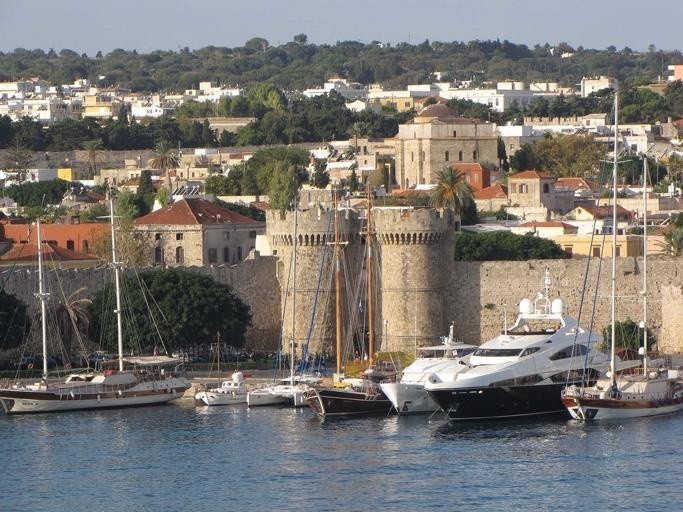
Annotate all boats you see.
[378,264,607,427]
[192,370,249,408]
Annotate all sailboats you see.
[244,186,394,422]
[0,193,192,417]
[558,84,683,424]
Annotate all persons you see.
[64,360,72,370]
[606,368,611,378]
[27,361,33,370]
